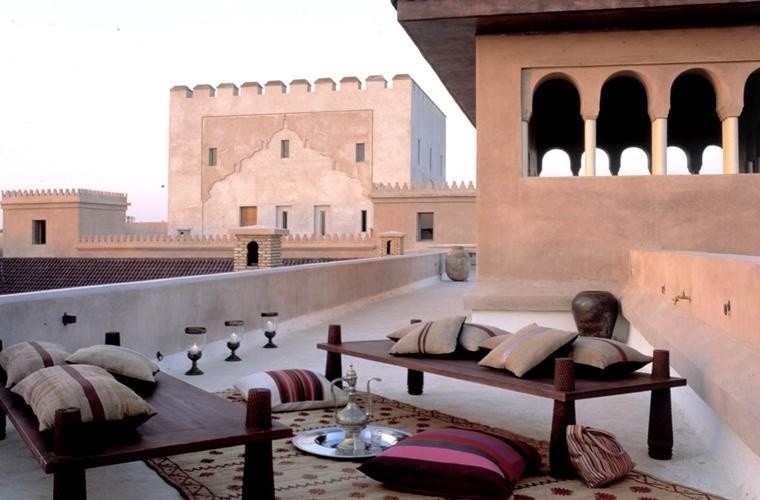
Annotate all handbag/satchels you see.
[566,424,637,488]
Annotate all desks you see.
[317,325,687,480]
[0,332,293,500]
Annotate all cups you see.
[352,431,383,454]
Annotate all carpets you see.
[141,386,728,500]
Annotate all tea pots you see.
[330,364,382,449]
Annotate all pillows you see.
[233,369,349,412]
[477,334,512,350]
[10,364,159,432]
[0,341,72,390]
[64,345,160,386]
[478,323,582,379]
[387,316,467,358]
[458,323,510,352]
[386,321,425,342]
[569,336,654,381]
[355,423,541,500]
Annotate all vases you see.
[568,289,620,339]
